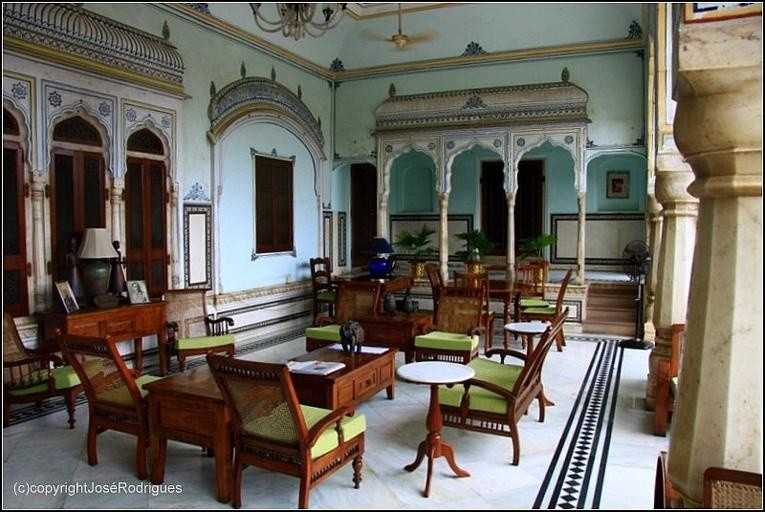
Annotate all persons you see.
[60,285,78,313]
[130,282,149,303]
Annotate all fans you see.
[367,2,442,55]
[618,240,656,350]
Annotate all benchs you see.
[427,306,569,471]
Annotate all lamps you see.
[368,236,393,283]
[80,226,120,294]
[247,3,353,40]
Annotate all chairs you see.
[307,255,577,372]
[53,322,166,481]
[654,323,686,438]
[160,286,236,372]
[203,349,369,505]
[653,447,763,510]
[2,312,108,428]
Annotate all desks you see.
[138,369,238,506]
[32,296,168,390]
[398,360,476,500]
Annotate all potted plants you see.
[520,229,555,281]
[451,228,491,288]
[392,227,442,279]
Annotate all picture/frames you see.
[53,280,80,314]
[125,278,151,305]
[607,169,630,199]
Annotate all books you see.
[284,360,346,376]
